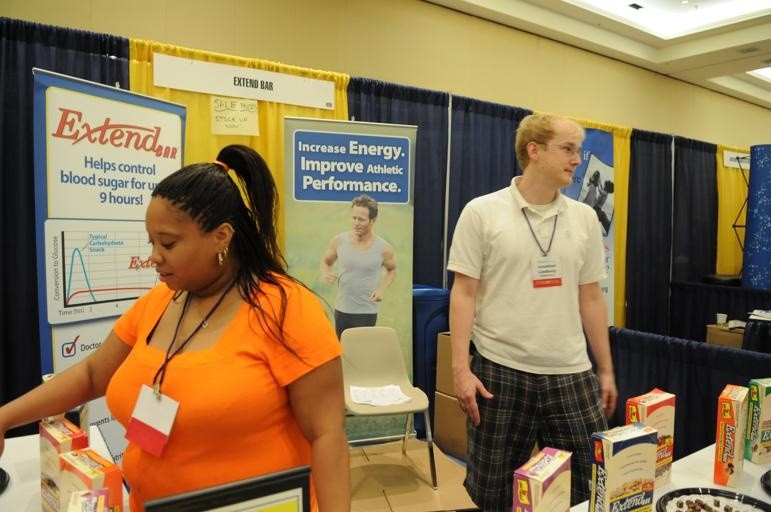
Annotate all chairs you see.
[339,325,438,491]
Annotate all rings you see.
[460,402,465,407]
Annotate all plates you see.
[655,487,771,512]
[760,469,771,494]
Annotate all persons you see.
[593,175,615,212]
[316,194,400,344]
[0,142,353,512]
[583,169,600,210]
[448,111,621,509]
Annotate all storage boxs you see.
[435,331,474,464]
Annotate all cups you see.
[717,313,728,325]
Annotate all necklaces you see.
[188,277,238,329]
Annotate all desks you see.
[572,427,771,511]
[0,423,143,512]
